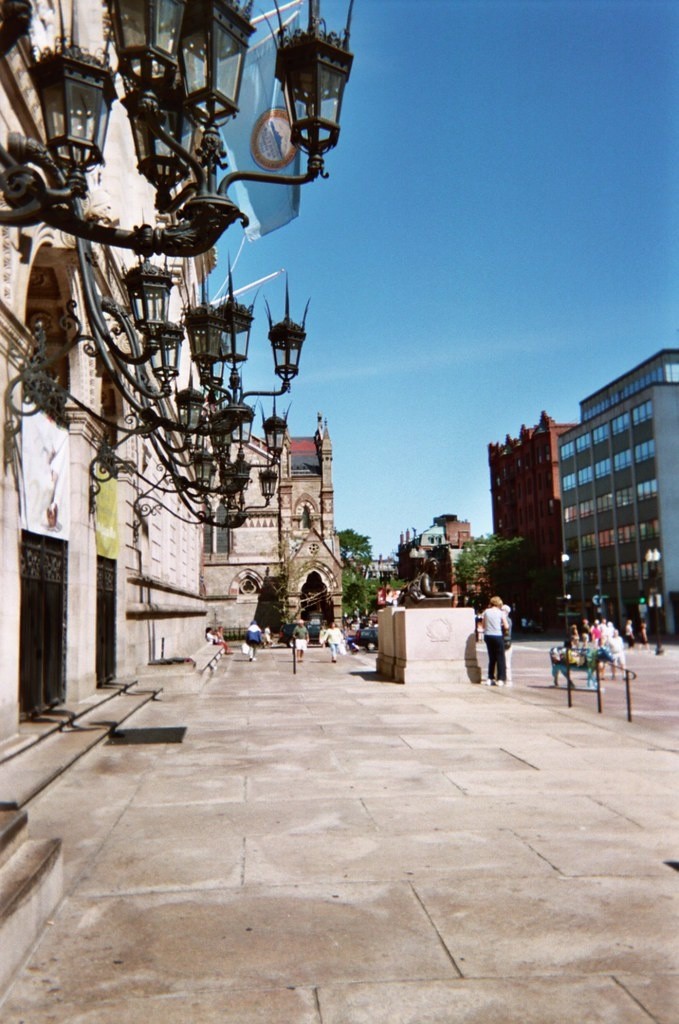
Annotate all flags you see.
[218,13,300,243]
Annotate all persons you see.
[482,596,512,688]
[318,622,358,663]
[207,626,233,655]
[565,617,649,681]
[246,620,275,662]
[405,558,454,602]
[293,619,310,662]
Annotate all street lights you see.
[645,548,664,655]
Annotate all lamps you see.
[0,0,354,534]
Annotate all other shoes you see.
[500,680,513,685]
[484,679,502,689]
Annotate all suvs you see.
[278,622,322,648]
[356,627,379,651]
